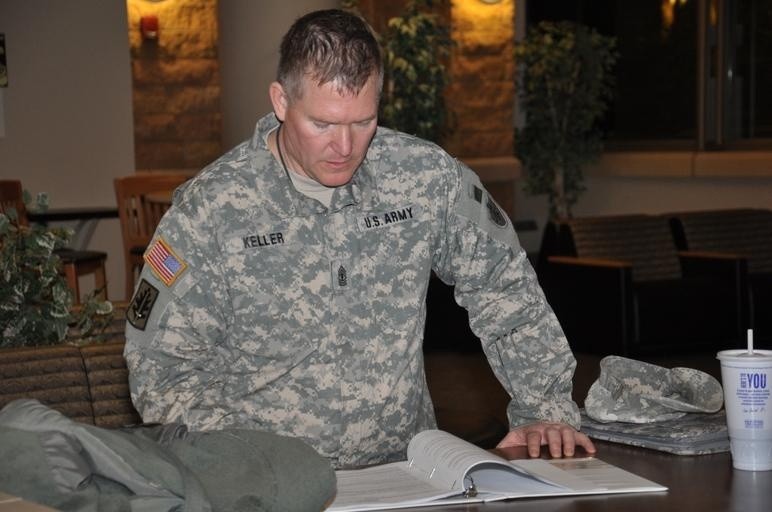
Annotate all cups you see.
[729,472,771,512]
[715,347,771,471]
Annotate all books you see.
[325,429,669,512]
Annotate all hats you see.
[583,354,725,423]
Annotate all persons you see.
[122,9,598,471]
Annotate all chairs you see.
[0,176,110,311]
[533,203,753,362]
[0,344,95,430]
[78,340,141,430]
[663,206,771,352]
[117,168,186,305]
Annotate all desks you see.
[326,415,772,511]
